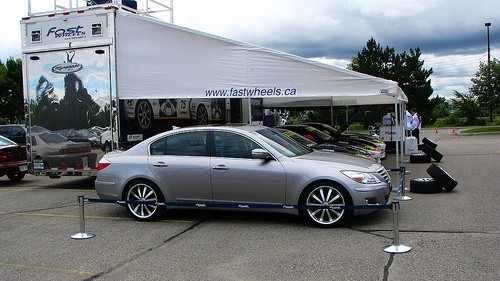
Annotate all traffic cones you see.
[452,129,455,133]
[436,128,438,134]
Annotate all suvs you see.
[0,124,27,146]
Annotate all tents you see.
[115,9,409,195]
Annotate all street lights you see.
[485,22,492,122]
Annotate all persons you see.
[382,108,394,125]
[410,108,419,143]
[405,106,413,136]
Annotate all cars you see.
[0,135,29,182]
[123,97,225,131]
[27,125,116,170]
[272,110,386,166]
[95,123,393,229]
